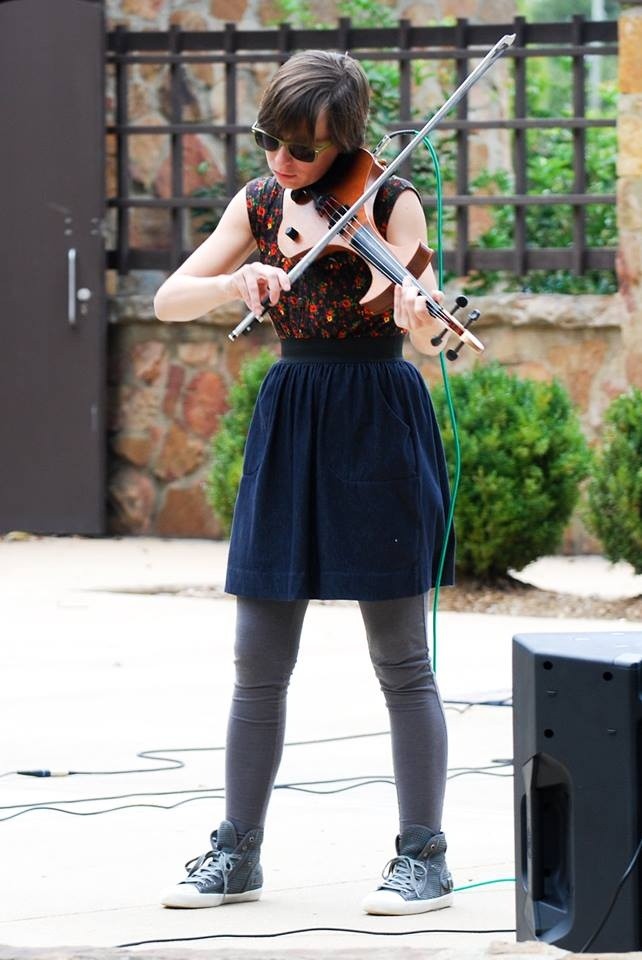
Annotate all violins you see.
[274,145,482,364]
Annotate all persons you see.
[153,43,460,921]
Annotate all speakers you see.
[513,632,641,953]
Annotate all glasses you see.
[251,119,336,165]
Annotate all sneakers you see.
[363,820,455,917]
[160,819,264,910]
